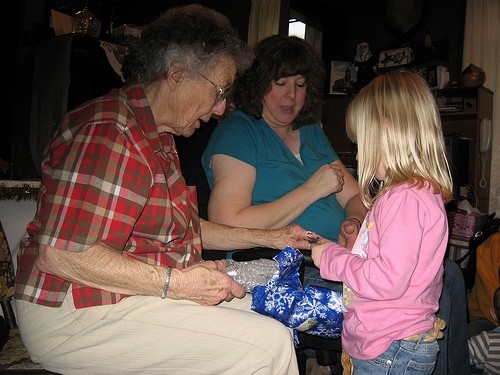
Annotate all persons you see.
[353,42,377,91]
[15,3,320,375]
[310,68,452,375]
[201,34,470,375]
[468,226,500,375]
[380,49,407,66]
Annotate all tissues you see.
[452,199,489,238]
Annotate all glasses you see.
[197,71,233,107]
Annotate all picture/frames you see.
[327,59,361,96]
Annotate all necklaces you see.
[283,125,289,139]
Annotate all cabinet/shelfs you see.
[28,31,146,180]
[322,85,494,220]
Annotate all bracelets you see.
[161,266,171,299]
[348,217,362,227]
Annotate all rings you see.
[340,177,342,180]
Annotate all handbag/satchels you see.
[449,210,500,287]
